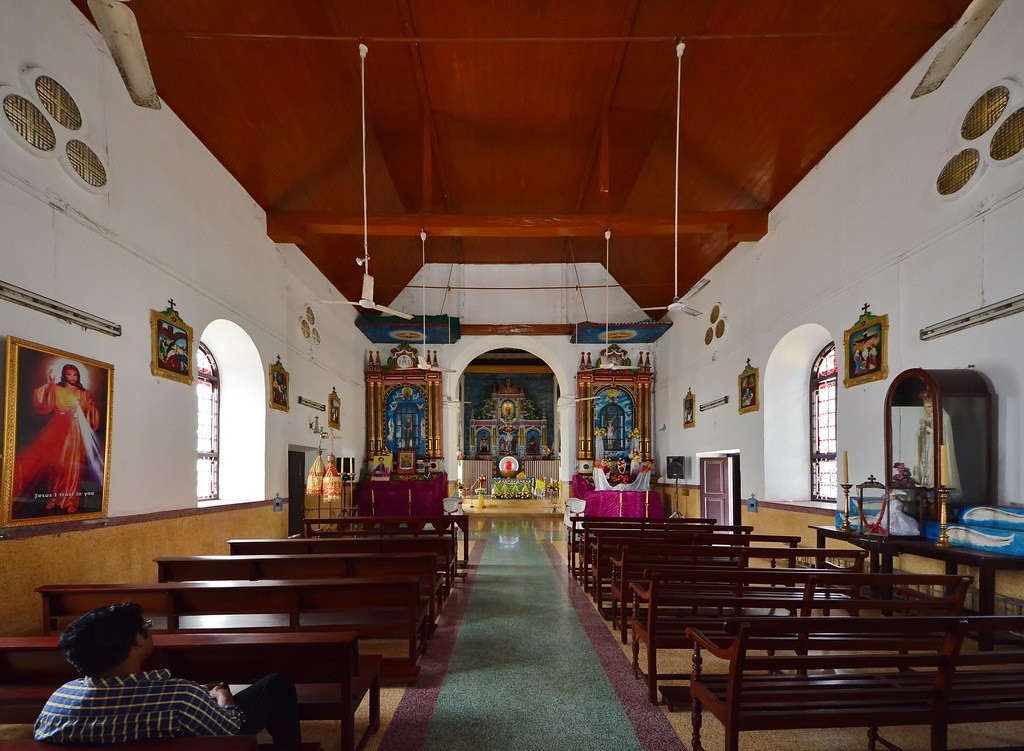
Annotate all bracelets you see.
[216,681,230,692]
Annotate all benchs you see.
[567,516,1024,751]
[0,514,470,751]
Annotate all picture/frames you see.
[0,336,114,527]
[328,392,341,429]
[151,309,193,385]
[268,362,290,413]
[843,314,889,387]
[682,395,696,429]
[738,366,759,413]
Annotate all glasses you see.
[140,620,153,630]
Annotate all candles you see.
[408,488,411,503]
[645,489,648,504]
[620,490,622,504]
[341,457,344,473]
[372,489,374,503]
[940,443,947,485]
[843,450,848,485]
[350,456,352,474]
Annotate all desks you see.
[882,539,1024,650]
[808,526,884,593]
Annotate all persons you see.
[34,600,302,744]
[611,457,628,483]
[873,496,920,535]
[602,455,611,479]
[373,458,389,477]
[401,455,411,468]
[503,430,516,442]
[915,402,960,489]
[629,447,641,477]
[14,365,99,511]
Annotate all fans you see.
[444,289,471,404]
[586,231,639,372]
[88,0,161,110]
[634,41,710,316]
[320,44,414,321]
[394,231,455,372]
[560,323,601,404]
[910,0,1004,98]
